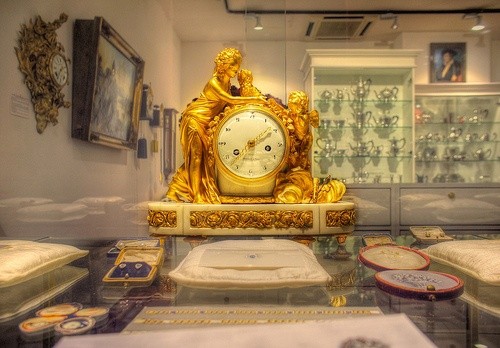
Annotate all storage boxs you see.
[102,240,165,288]
[18,302,110,342]
[357,226,464,302]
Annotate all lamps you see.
[253,15,263,30]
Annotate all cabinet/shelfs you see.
[300,48,500,235]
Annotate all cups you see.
[321,120,330,126]
[333,119,344,126]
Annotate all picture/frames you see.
[71,15,179,178]
[429,43,466,84]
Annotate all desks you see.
[0,236,500,348]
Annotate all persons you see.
[162,48,269,203]
[270,92,313,171]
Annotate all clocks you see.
[15,12,72,135]
[147,47,357,236]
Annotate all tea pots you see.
[415,110,498,160]
[349,74,407,155]
[316,136,339,156]
